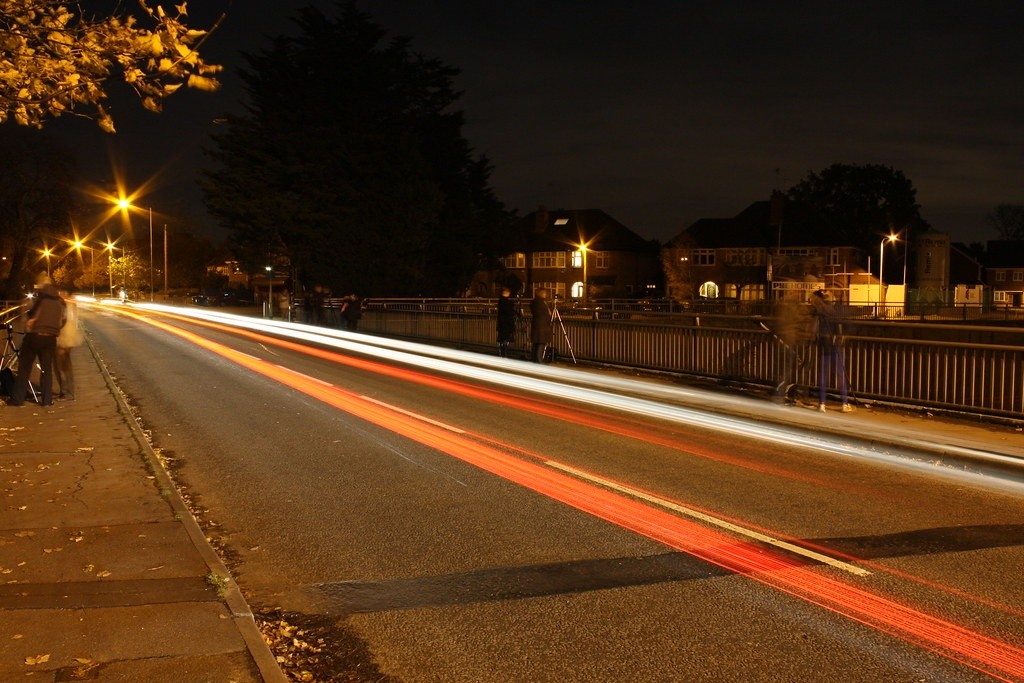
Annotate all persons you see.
[6,274,82,406]
[808,286,857,415]
[496,287,517,357]
[529,288,553,364]
[279,277,365,332]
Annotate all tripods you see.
[511,297,533,357]
[0,324,39,404]
[541,298,578,364]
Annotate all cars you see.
[191,291,240,306]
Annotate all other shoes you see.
[42,401,54,407]
[10,399,25,407]
[56,394,74,401]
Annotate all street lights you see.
[105,243,114,296]
[44,250,51,278]
[878,235,898,306]
[579,244,587,303]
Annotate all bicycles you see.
[0,322,45,397]
